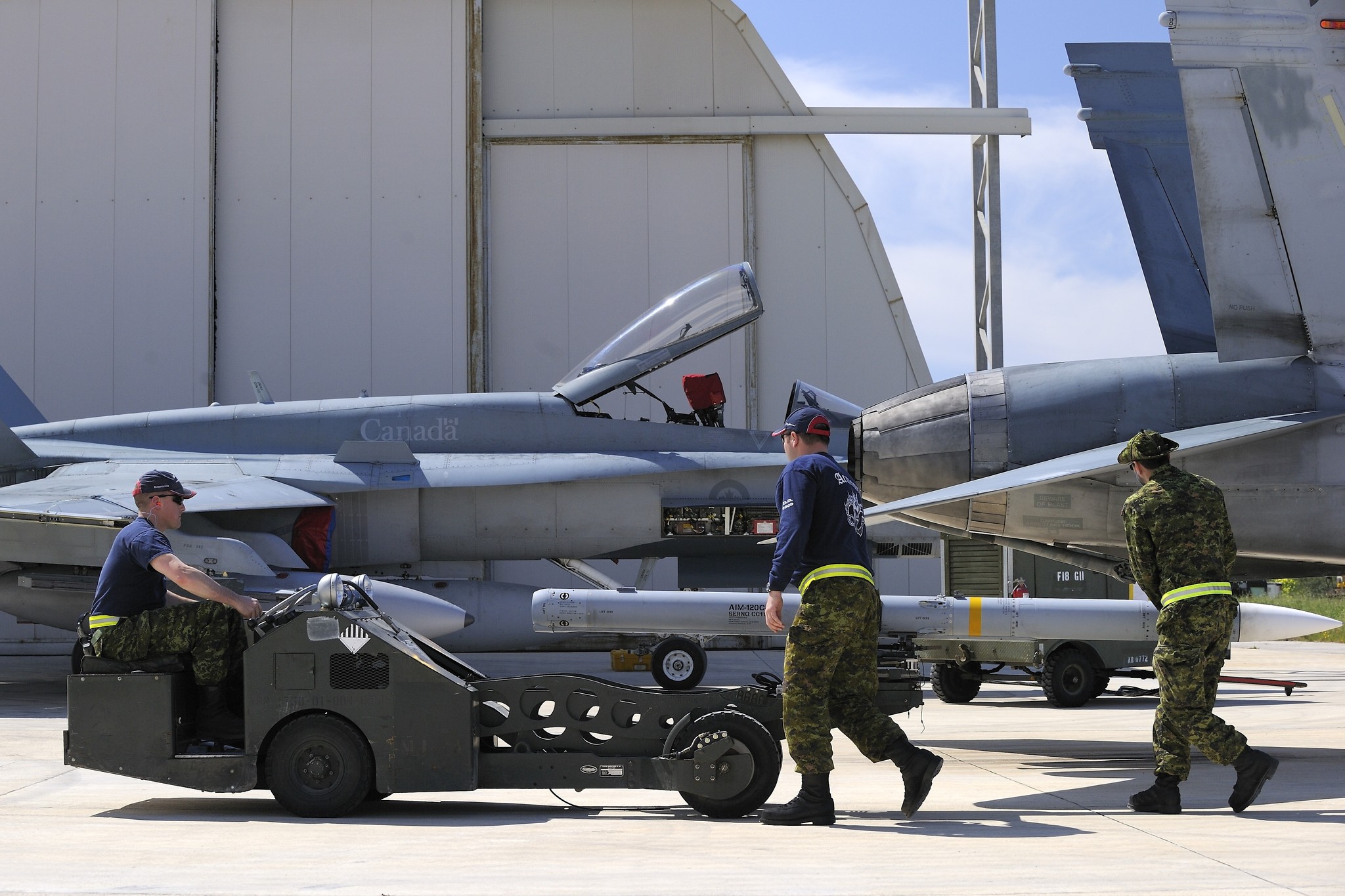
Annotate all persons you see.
[756,407,944,827]
[90,470,263,749]
[1115,428,1280,814]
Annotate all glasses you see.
[149,494,184,506]
[1128,460,1141,471]
[780,431,799,445]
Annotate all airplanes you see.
[0,1,1345,638]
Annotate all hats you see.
[772,406,830,437]
[132,469,197,499]
[1117,429,1179,464]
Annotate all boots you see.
[1228,744,1279,813]
[1126,772,1182,814]
[193,685,245,739]
[882,736,944,819]
[759,772,835,826]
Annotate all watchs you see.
[766,582,772,592]
